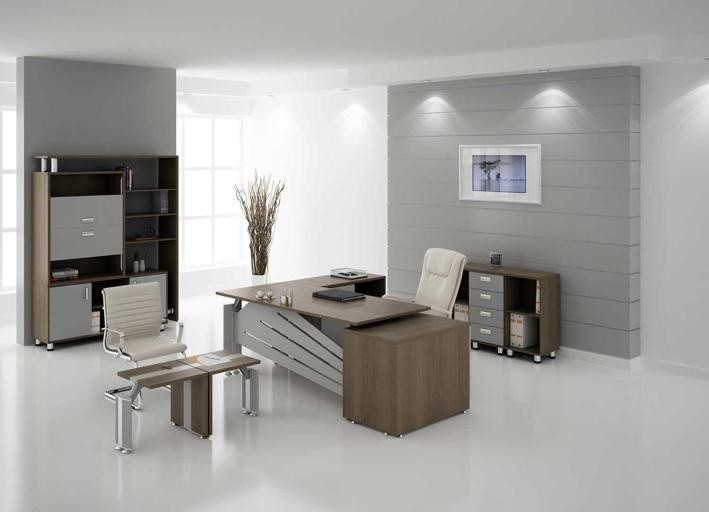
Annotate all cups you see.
[280,288,294,306]
[132,261,139,272]
[140,260,146,272]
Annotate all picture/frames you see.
[458,144,542,205]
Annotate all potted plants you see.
[232,167,287,285]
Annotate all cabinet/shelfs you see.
[31,154,179,351]
[452,262,561,363]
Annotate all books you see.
[311,288,367,302]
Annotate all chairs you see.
[381,247,468,320]
[100,281,188,411]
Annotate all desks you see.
[104,349,261,454]
[215,272,472,438]
